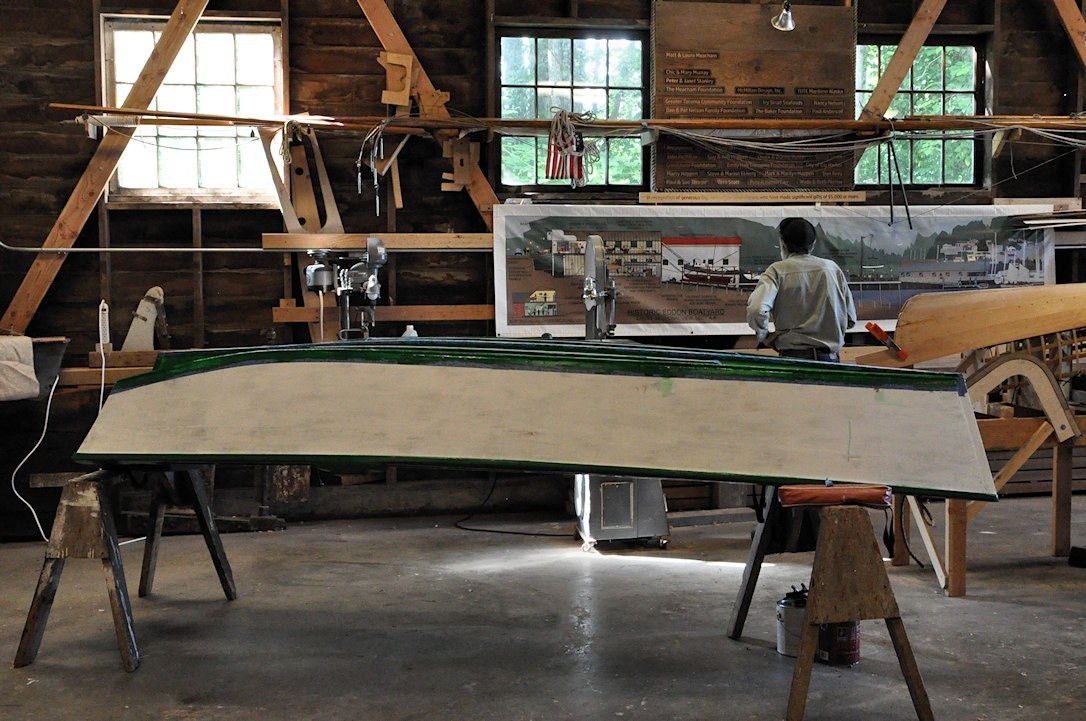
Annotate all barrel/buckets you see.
[818,620,860,666]
[776,597,807,657]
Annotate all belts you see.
[778,347,835,357]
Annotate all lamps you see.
[771,0,796,32]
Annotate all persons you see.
[747,218,857,553]
